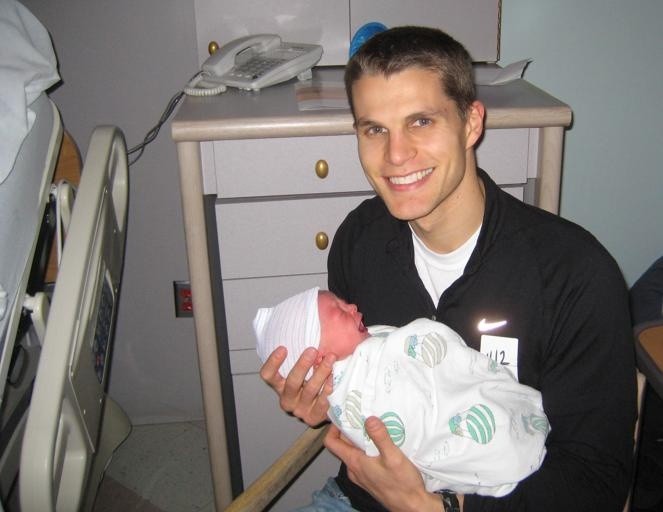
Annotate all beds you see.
[1,0,134,512]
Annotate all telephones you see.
[202,34,324,92]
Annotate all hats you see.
[252,285,321,388]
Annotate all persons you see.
[252,287,551,497]
[272,23,641,512]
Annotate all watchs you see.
[434,487,461,512]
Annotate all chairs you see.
[221,420,341,511]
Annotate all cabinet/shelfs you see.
[171,67,573,511]
